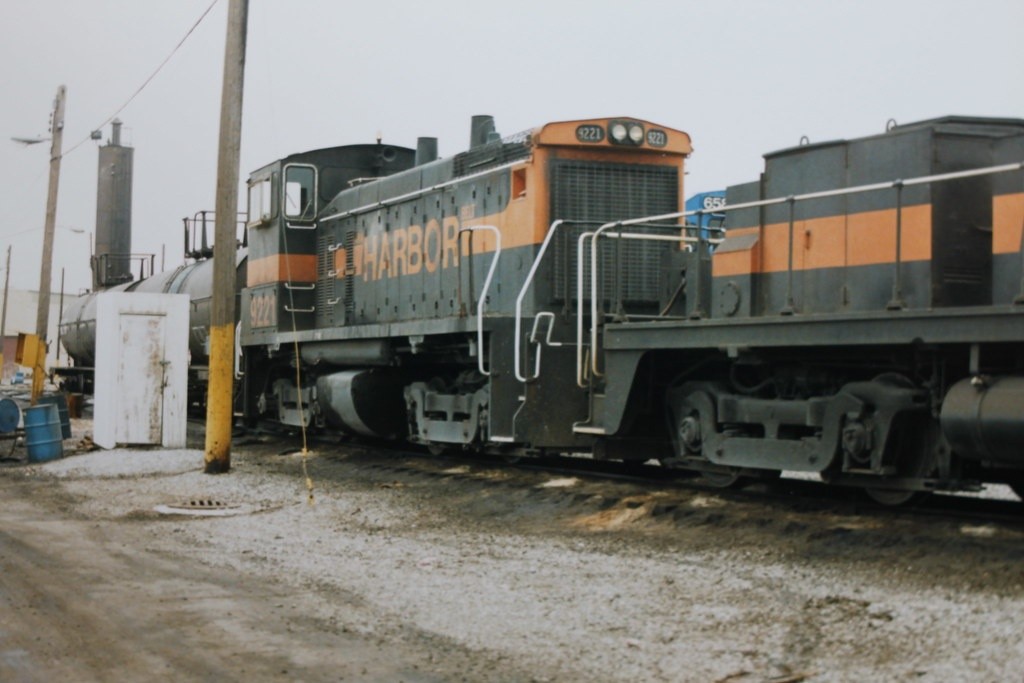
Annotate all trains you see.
[53,111,1024,521]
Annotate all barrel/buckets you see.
[37,395,71,439]
[27,403,63,459]
[0,399,19,432]
